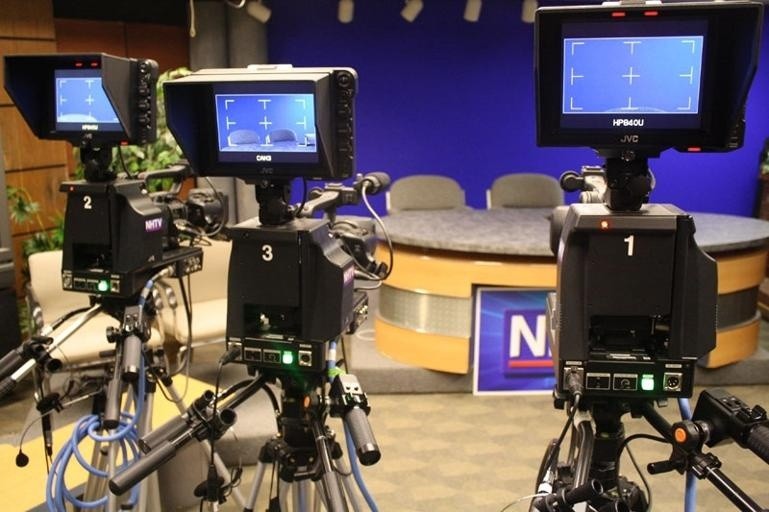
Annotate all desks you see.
[369,206,769,379]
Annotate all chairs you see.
[483,169,565,209]
[385,171,469,216]
[23,250,162,456]
[154,232,234,374]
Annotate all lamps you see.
[246,0,538,24]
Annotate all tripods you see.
[244,422,360,512]
[81,306,247,512]
